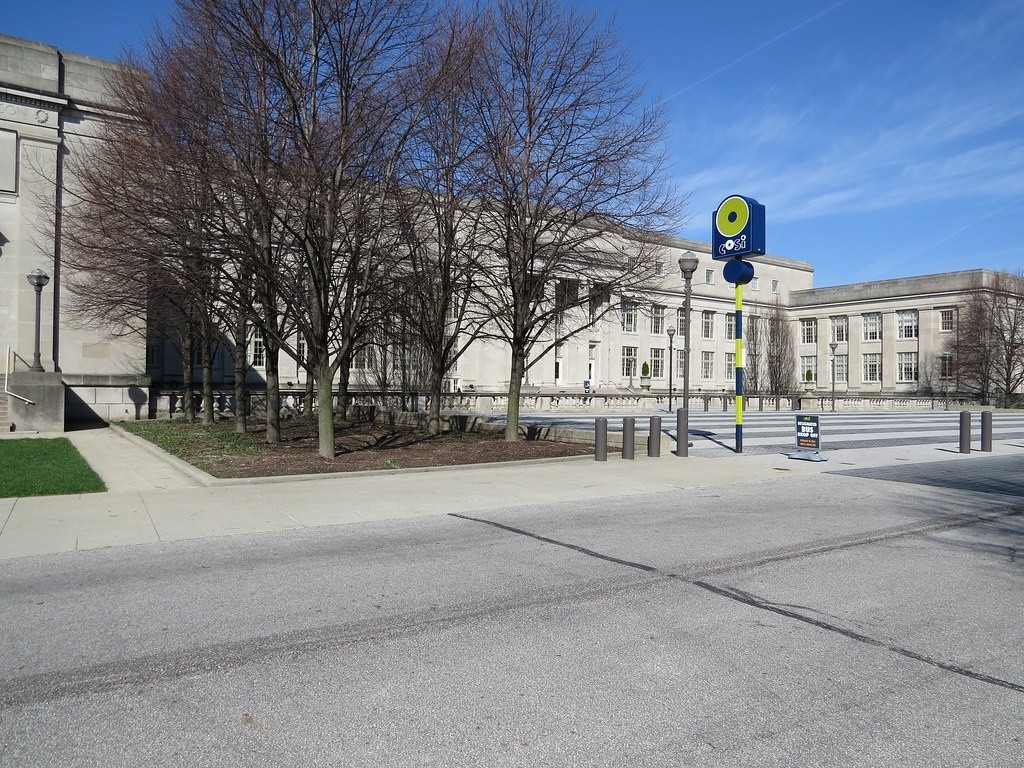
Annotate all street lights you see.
[829,340,838,412]
[667,324,676,412]
[679,251,699,409]
[944,351,950,412]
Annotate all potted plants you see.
[640,362,651,393]
[803,369,816,397]
